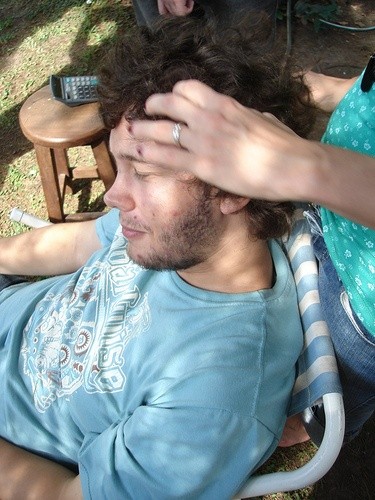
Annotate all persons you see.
[124,30,375,448]
[0,26,316,500]
[122,0,195,31]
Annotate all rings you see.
[173,121,186,150]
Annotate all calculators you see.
[50,73,102,108]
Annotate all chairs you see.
[232,191,345,500]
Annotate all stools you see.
[19,81,117,226]
